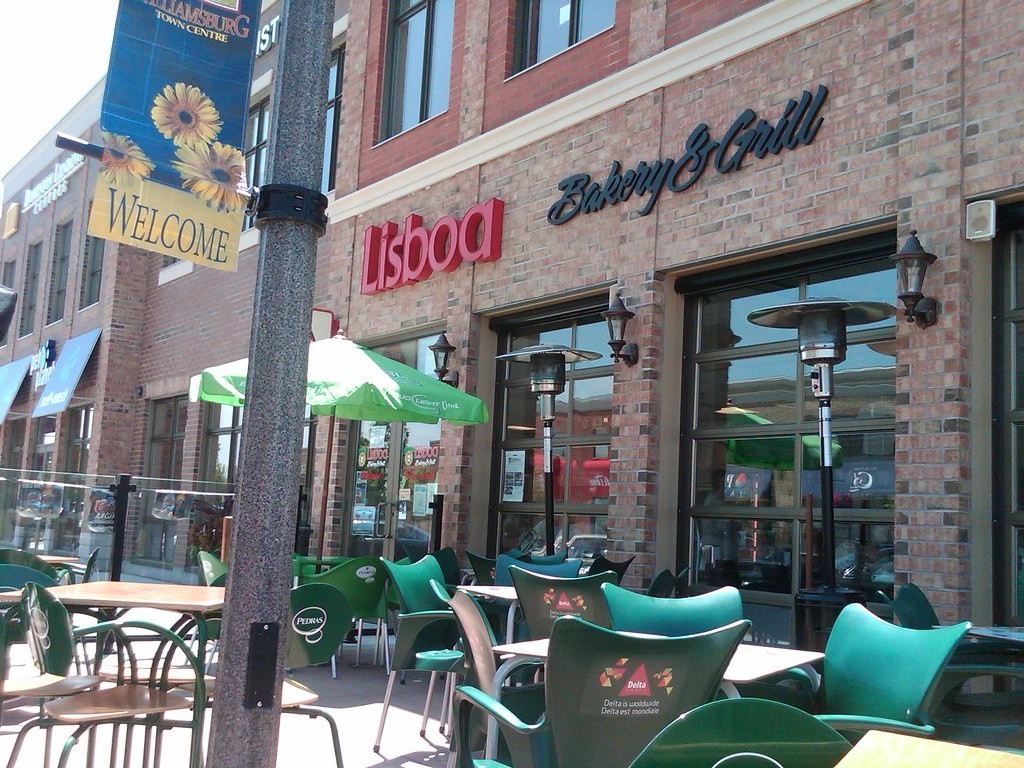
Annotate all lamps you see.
[600,293,639,368]
[428,333,459,387]
[888,230,938,329]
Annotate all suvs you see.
[531,523,606,561]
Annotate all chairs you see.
[0,546,1024,768]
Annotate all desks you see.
[32,555,78,583]
[458,586,522,646]
[0,580,224,768]
[931,627,1023,691]
[486,631,824,758]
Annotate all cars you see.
[351,520,430,558]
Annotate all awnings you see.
[31,329,101,419]
[0,355,33,426]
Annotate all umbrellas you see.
[188,329,492,574]
[723,403,843,562]
